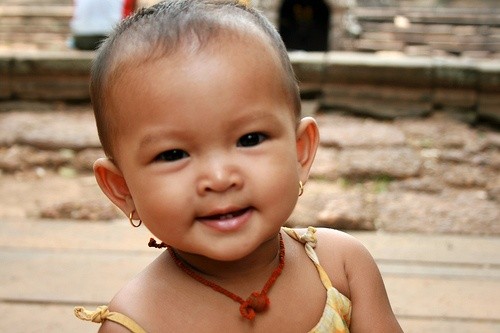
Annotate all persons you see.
[74,0,404,333]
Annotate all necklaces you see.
[147,230,287,322]
